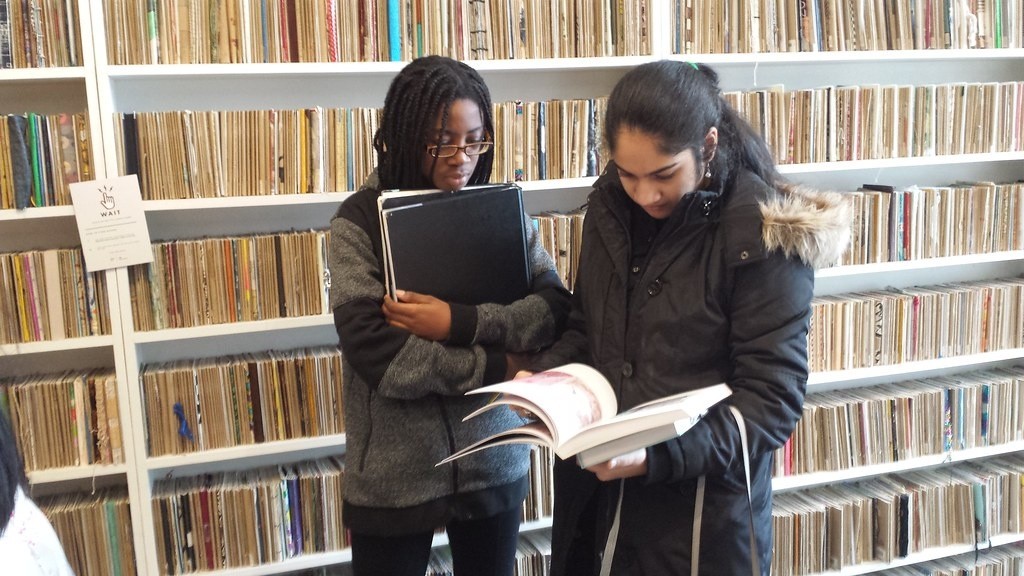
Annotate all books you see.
[0,0,1024,576]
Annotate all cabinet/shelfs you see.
[0,0,147,576]
[90,0,1024,576]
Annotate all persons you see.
[551,59,854,576]
[0,406,77,575]
[325,56,573,576]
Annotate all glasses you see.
[423,126,493,158]
